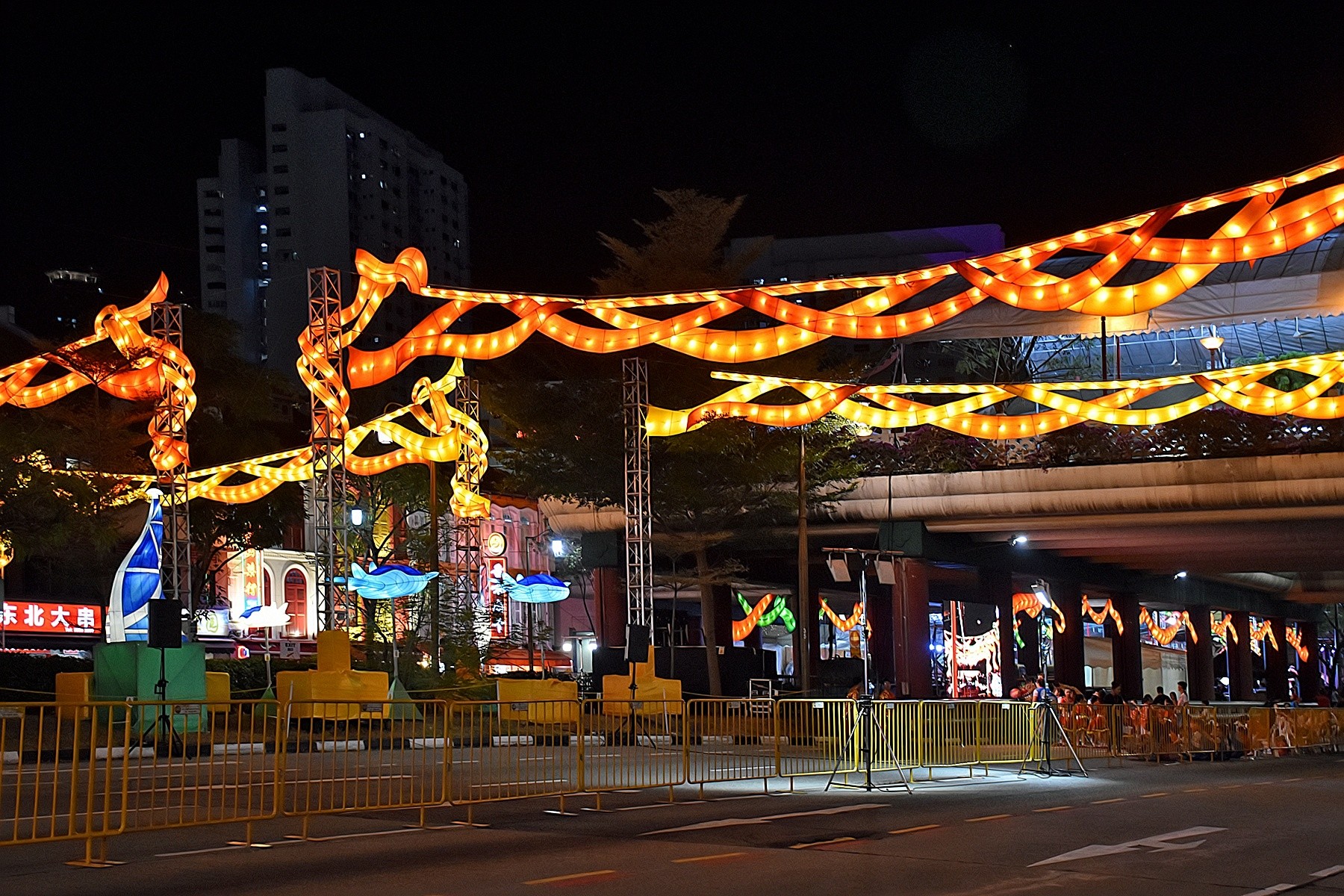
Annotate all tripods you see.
[129,650,192,760]
[1018,671,1086,777]
[824,699,913,796]
[618,662,656,749]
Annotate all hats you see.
[1178,681,1187,688]
[1111,681,1120,689]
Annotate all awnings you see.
[483,649,572,665]
[205,642,236,654]
[5,637,95,651]
[240,641,426,661]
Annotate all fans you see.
[1143,331,1200,368]
[1280,317,1315,340]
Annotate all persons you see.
[1288,665,1299,698]
[785,658,794,682]
[1032,672,1344,762]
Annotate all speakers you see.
[1016,606,1053,669]
[146,598,181,649]
[879,520,926,557]
[624,625,649,662]
